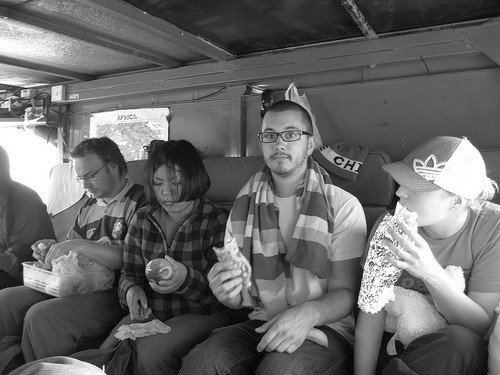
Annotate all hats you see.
[382,135,487,199]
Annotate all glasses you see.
[257,129,312,143]
[74,161,108,182]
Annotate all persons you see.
[98,140,244,375]
[0,137,149,364]
[354,136,500,375]
[0,146,59,290]
[178,100,367,375]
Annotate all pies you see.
[213,237,251,288]
[392,201,417,227]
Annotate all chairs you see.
[42,147,500,242]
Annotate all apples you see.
[145,257,174,284]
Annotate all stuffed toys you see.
[384,265,466,355]
